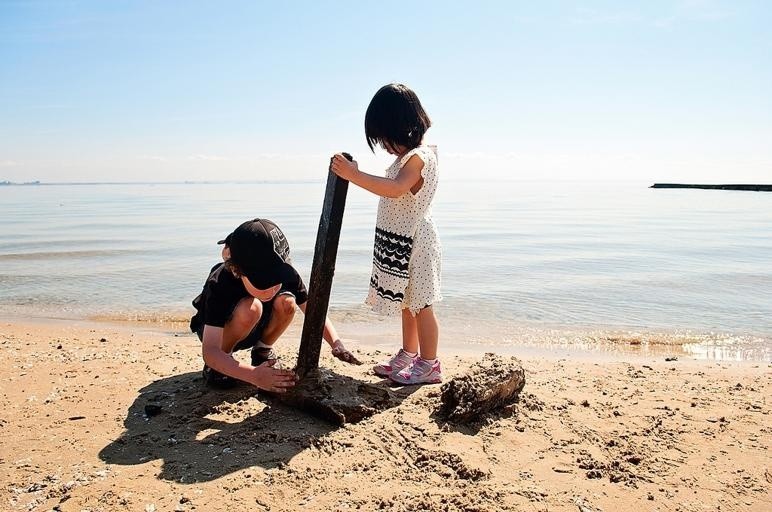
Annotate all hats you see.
[216,217,298,291]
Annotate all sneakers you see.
[372,349,420,377]
[202,363,240,390]
[389,357,442,385]
[250,346,280,368]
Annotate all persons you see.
[330,81,445,386]
[189,217,360,393]
